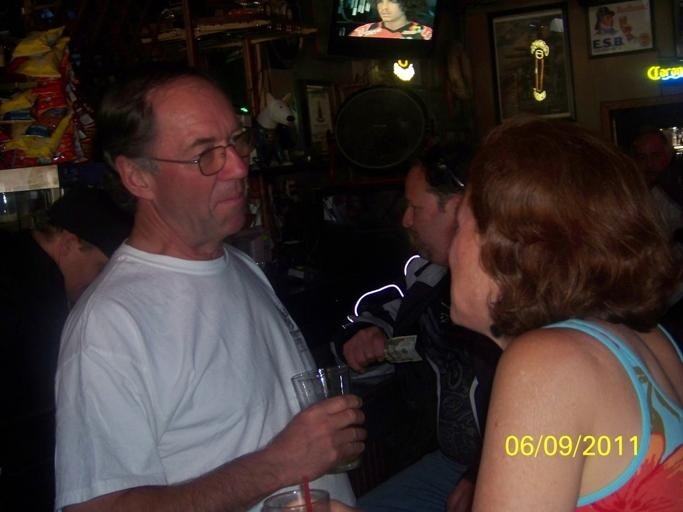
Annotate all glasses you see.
[137,129,252,175]
[429,145,464,187]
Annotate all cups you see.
[262,489,332,511]
[292,365,363,474]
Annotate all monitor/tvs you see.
[327,0,441,58]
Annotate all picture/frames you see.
[598,93,681,182]
[486,3,577,126]
[583,1,658,59]
[298,76,332,154]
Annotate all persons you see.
[622,127,680,352]
[46,60,369,512]
[327,142,506,509]
[0,187,133,509]
[442,111,681,509]
[347,0,432,40]
[591,7,619,36]
